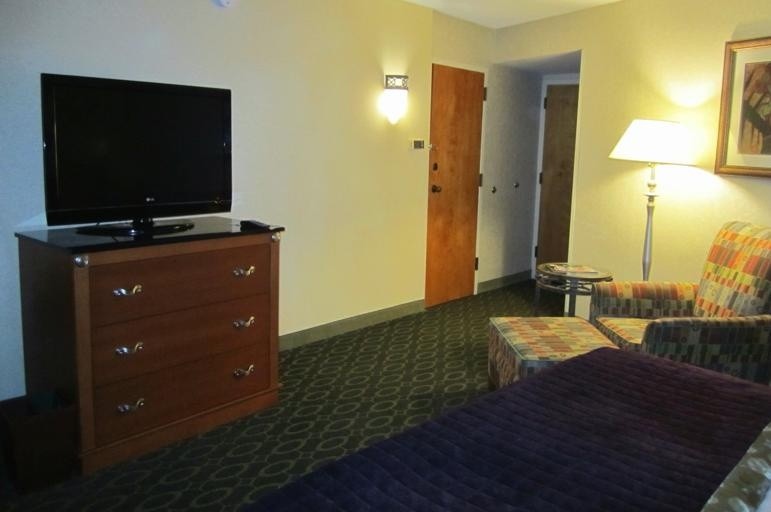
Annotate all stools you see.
[488,314,620,390]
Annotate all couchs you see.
[589,220,771,384]
[275,346,770,512]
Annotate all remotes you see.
[240,220,271,229]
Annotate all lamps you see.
[608,118,703,282]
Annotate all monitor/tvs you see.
[40,72,232,236]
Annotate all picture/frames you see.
[713,36,771,177]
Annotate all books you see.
[545,263,598,273]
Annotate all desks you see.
[13,215,279,477]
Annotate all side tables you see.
[532,262,615,317]
